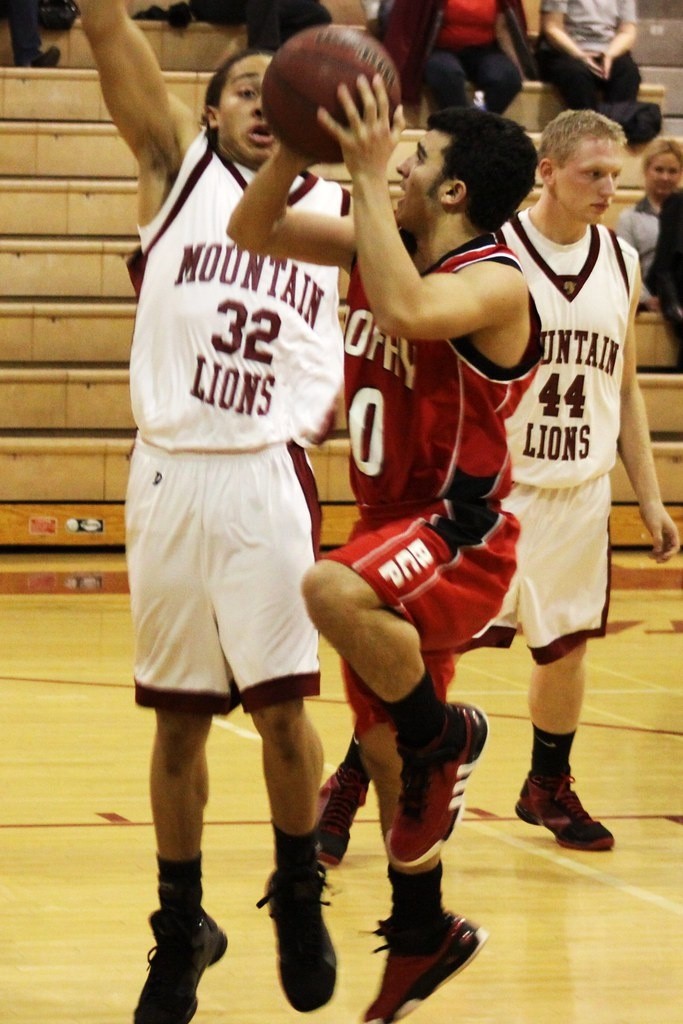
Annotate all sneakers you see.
[361,907,486,1024]
[133,903,228,1024]
[310,763,369,866]
[255,861,339,1013]
[385,702,491,874]
[516,771,614,849]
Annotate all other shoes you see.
[25,44,60,67]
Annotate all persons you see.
[615,140,683,373]
[227,25,545,1024]
[75,0,346,1024]
[317,111,680,867]
[0,0,60,67]
[246,0,663,146]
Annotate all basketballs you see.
[259,23,401,166]
